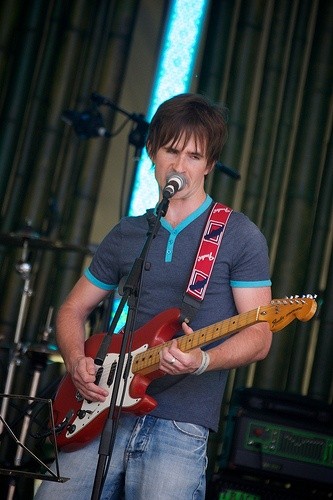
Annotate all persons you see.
[34,94,273,500]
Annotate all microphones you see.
[162,173,187,199]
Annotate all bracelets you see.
[193,351,210,375]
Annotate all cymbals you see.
[0,229,95,255]
[0,338,61,354]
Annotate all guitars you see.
[49,293,318,453]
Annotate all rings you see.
[170,358,177,366]
